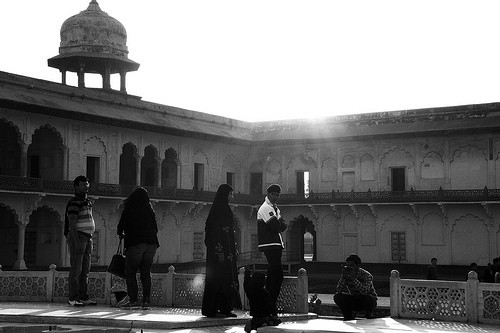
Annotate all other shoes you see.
[223,310,238,319]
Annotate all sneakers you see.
[140,301,152,311]
[122,300,138,309]
[67,300,84,307]
[84,299,98,306]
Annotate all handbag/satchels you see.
[106,253,127,279]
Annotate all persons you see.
[202,184,238,317]
[116,186,159,309]
[426,258,500,312]
[257,184,288,315]
[333,254,378,322]
[64,176,96,306]
[243,265,282,333]
